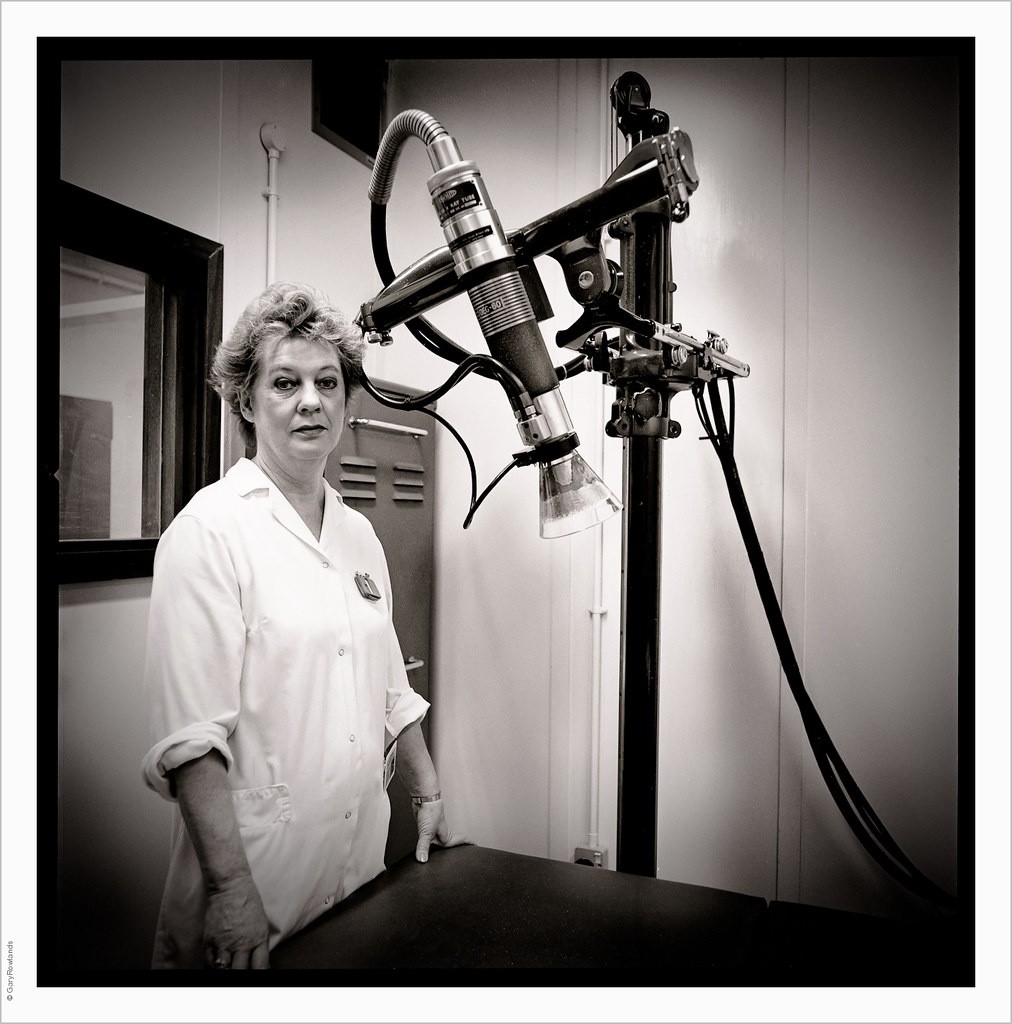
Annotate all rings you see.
[215,959,227,968]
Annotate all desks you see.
[248,841,957,968]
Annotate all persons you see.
[143,283,465,969]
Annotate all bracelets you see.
[410,791,441,803]
